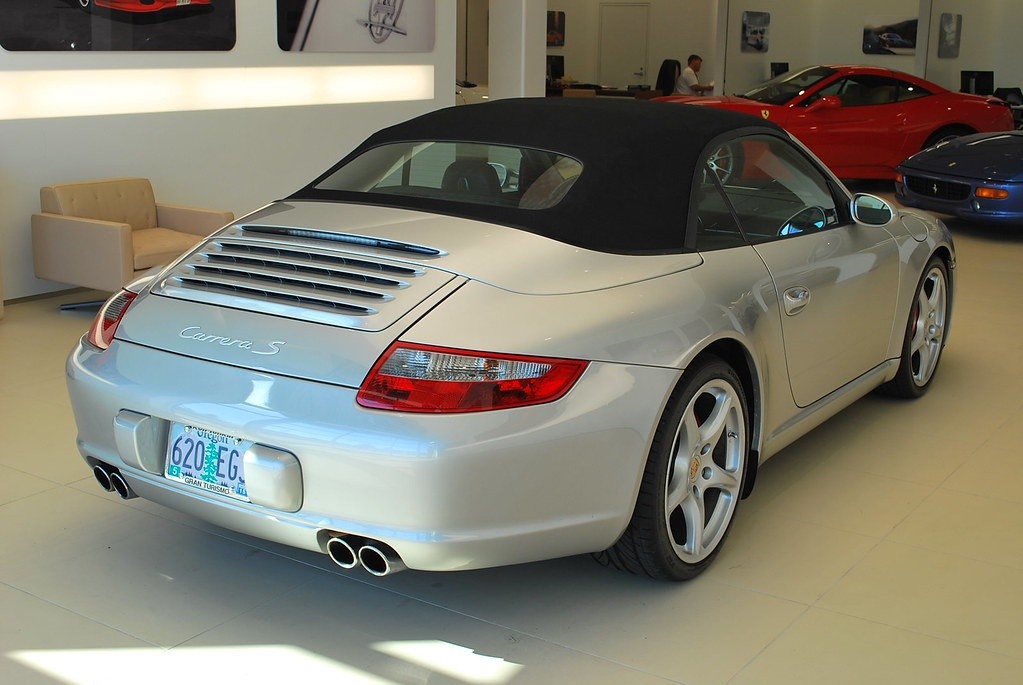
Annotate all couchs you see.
[29,178,234,312]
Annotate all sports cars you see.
[76,1,211,16]
[62,95,958,585]
[648,66,1016,180]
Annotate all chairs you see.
[442,150,506,204]
[655,58,681,93]
[837,84,903,106]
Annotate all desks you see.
[551,86,662,98]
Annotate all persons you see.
[676,54,714,96]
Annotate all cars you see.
[892,127,1023,229]
